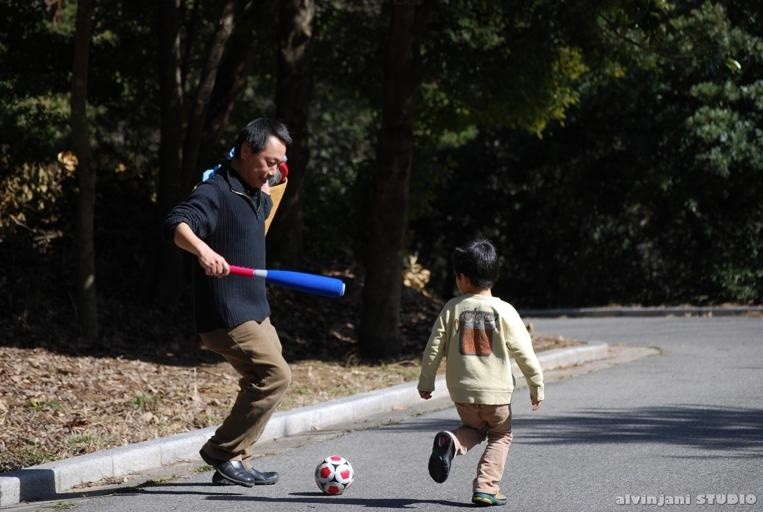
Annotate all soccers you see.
[315,455,355,494]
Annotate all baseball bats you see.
[228,266,346,299]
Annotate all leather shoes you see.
[198,442,255,488]
[212,465,279,487]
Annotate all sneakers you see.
[472,491,507,506]
[427,431,455,484]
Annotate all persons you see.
[416,237,544,505]
[163,114,292,486]
